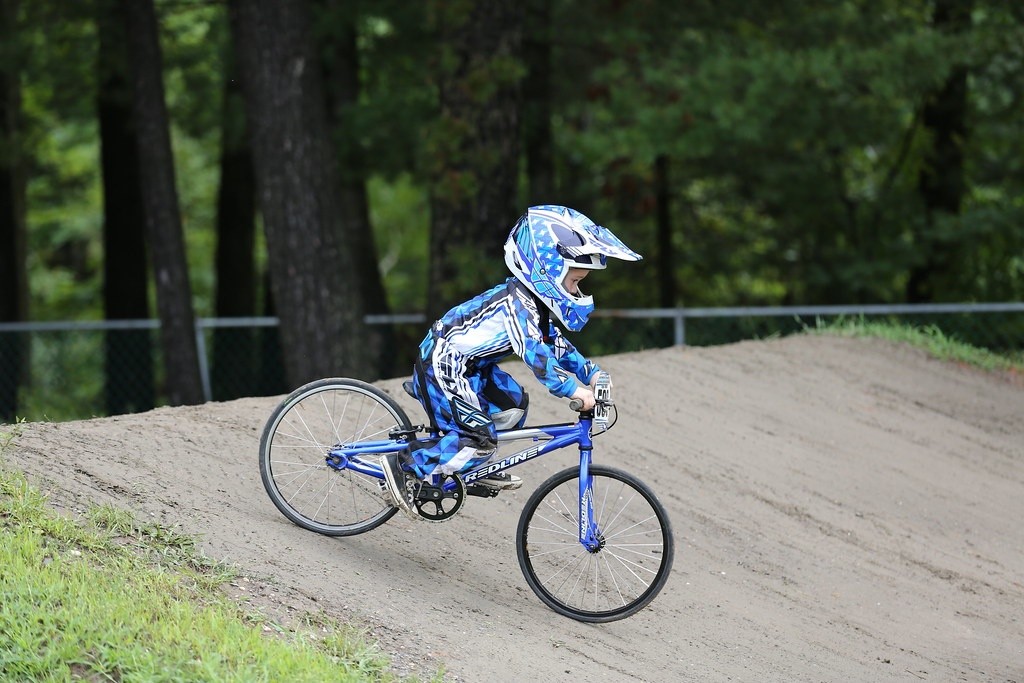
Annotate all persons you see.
[379,204,643,519]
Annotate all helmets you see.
[504,205,644,332]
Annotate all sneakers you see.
[455,461,524,490]
[379,452,419,522]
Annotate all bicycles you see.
[258,377,675,624]
